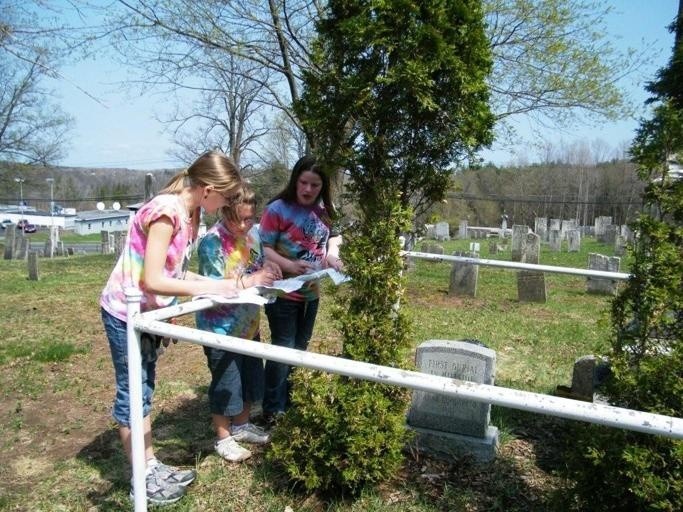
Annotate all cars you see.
[0,219,36,233]
[48,207,61,216]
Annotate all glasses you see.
[215,190,237,205]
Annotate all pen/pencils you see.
[237,272,241,288]
[250,262,279,278]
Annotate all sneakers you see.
[130,473,186,506]
[150,459,195,487]
[214,438,252,462]
[229,421,270,444]
[264,411,277,425]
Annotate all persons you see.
[195,182,284,463]
[256,154,349,426]
[96,149,243,505]
